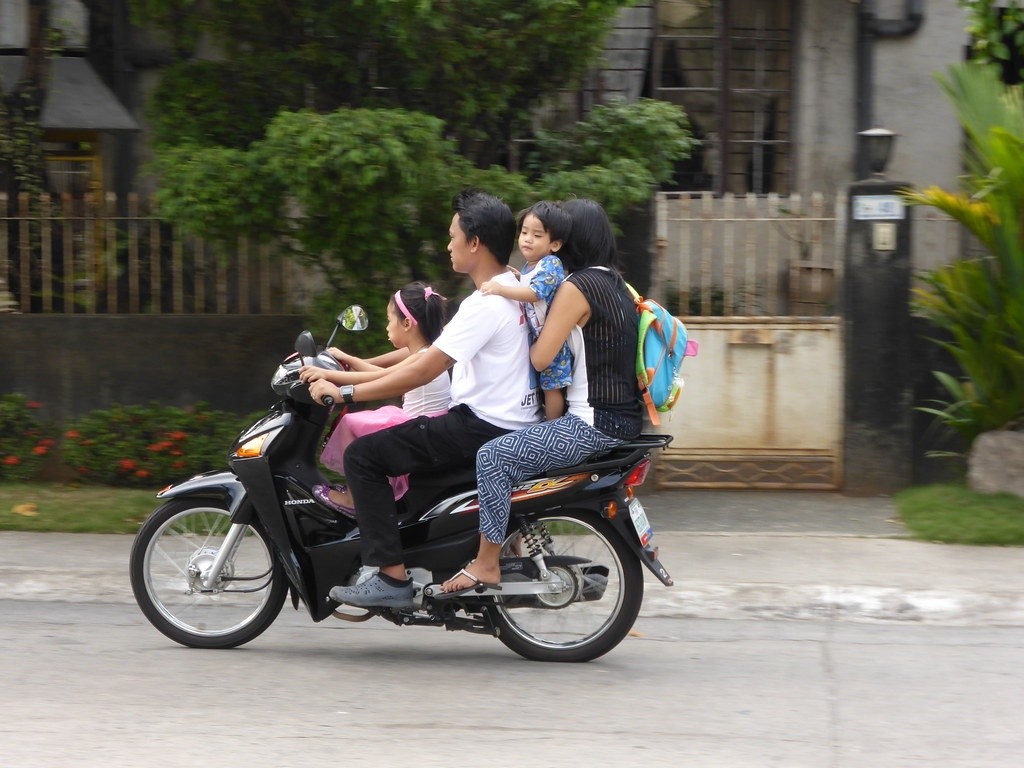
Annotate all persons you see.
[481,201,572,420]
[310,189,544,607]
[298,281,452,520]
[435,199,641,600]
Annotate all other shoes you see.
[312,483,358,520]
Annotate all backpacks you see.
[562,265,699,427]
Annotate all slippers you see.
[434,568,503,600]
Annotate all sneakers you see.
[328,575,414,609]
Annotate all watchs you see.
[339,384,354,404]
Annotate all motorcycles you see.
[127,304,675,661]
